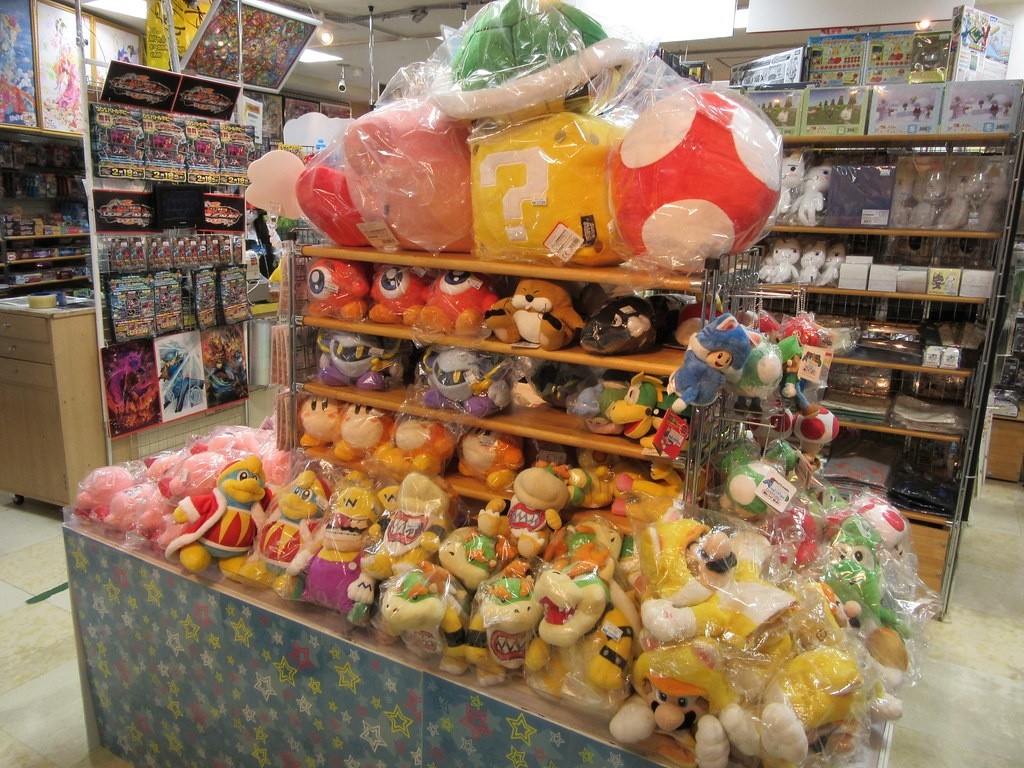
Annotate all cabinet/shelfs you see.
[278,226,765,520]
[764,133,1024,626]
[0,232,90,294]
[0,295,139,508]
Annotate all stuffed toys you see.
[667,312,839,472]
[777,152,1008,231]
[302,257,722,354]
[66,399,914,768]
[758,237,846,287]
[246,0,782,273]
[302,326,713,460]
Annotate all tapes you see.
[27,293,57,309]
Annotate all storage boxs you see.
[728,1,1024,136]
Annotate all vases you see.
[244,89,283,141]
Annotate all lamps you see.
[460,4,469,26]
[317,27,333,46]
[411,8,429,23]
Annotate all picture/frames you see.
[320,103,352,119]
[0,0,45,134]
[283,97,320,142]
[38,0,143,140]
[181,0,323,95]
